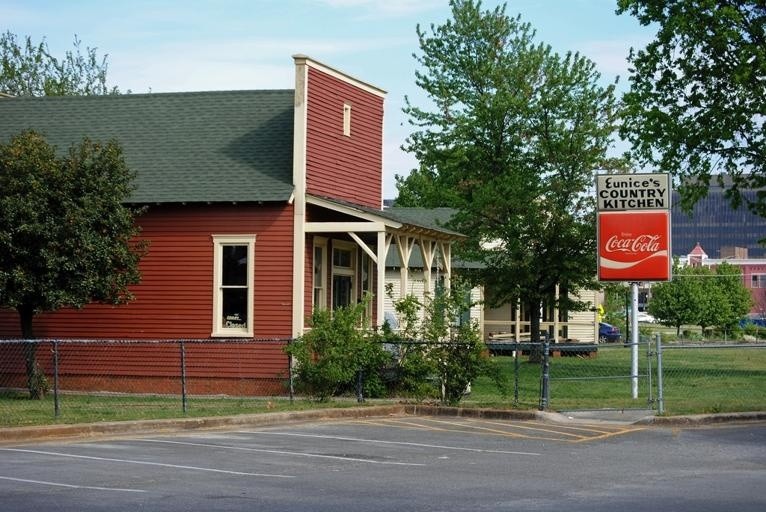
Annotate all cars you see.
[598,317,622,344]
[637,312,657,324]
